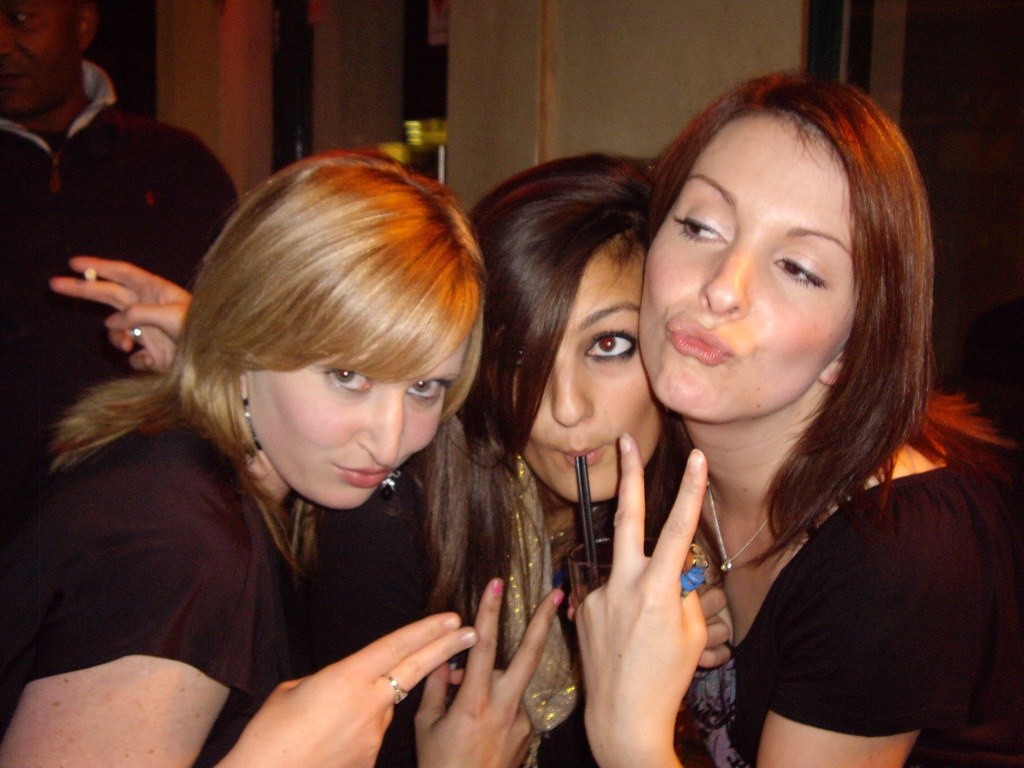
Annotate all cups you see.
[566,537,700,760]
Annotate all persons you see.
[0,151,478,768]
[50,66,1024,768]
[384,152,746,768]
[0,0,240,552]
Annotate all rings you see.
[385,674,409,704]
[130,327,142,339]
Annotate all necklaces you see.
[707,480,769,571]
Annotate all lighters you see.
[680,544,709,600]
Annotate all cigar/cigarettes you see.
[84,268,97,280]
